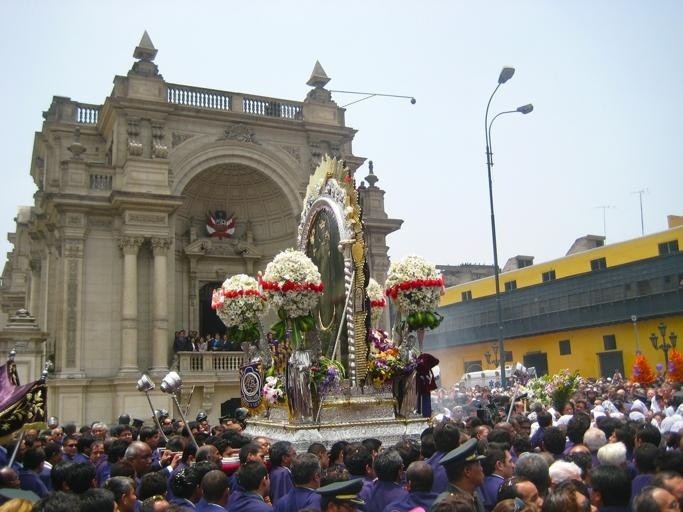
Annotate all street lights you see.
[630,190,646,236]
[649,322,678,371]
[487,67,535,392]
[483,342,508,372]
[596,204,615,245]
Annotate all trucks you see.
[453,368,510,391]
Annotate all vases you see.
[282,345,312,425]
[390,334,420,419]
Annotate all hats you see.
[515,392,529,402]
[437,436,488,467]
[315,478,368,507]
[76,437,94,454]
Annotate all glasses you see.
[513,497,525,512]
[65,443,77,448]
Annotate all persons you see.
[174,328,243,351]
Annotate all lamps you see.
[134,369,172,449]
[157,369,202,451]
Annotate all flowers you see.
[215,275,269,347]
[363,323,406,391]
[361,278,388,322]
[545,367,581,412]
[257,245,325,353]
[526,371,553,405]
[308,353,345,400]
[259,357,286,407]
[384,252,446,333]
